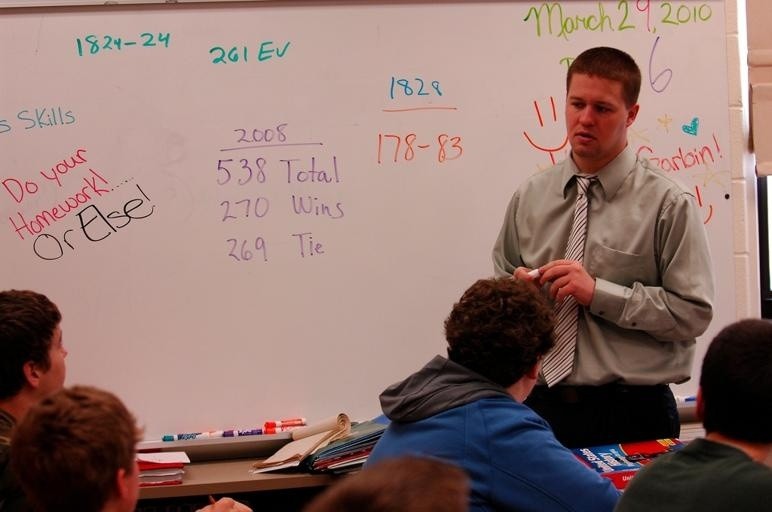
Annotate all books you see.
[571,433,682,491]
[249,413,392,478]
[135,447,192,487]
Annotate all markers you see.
[162,417,307,441]
[684,396,696,401]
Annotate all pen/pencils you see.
[208,494,216,504]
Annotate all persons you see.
[0,287,69,433]
[495,46,715,448]
[1,383,257,512]
[302,457,474,512]
[613,316,771,511]
[361,274,624,511]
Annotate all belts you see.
[528,384,670,396]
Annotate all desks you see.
[134,430,366,503]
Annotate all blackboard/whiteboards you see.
[0,0,760,445]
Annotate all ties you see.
[541,176,592,389]
